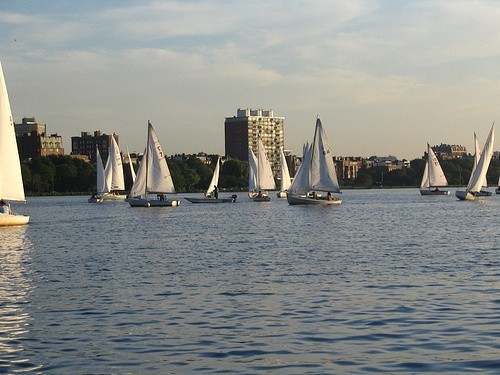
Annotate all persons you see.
[326,191,333,201]
[257,190,264,198]
[0,199,13,215]
[213,185,219,199]
[157,194,165,201]
[434,186,440,192]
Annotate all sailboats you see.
[466,131,493,196]
[286,113,344,206]
[128,119,181,207]
[184,157,238,204]
[419,143,451,195]
[247,137,277,201]
[277,147,291,198]
[455,120,496,201]
[0,61,31,227]
[88,134,137,204]
[495,177,500,194]
